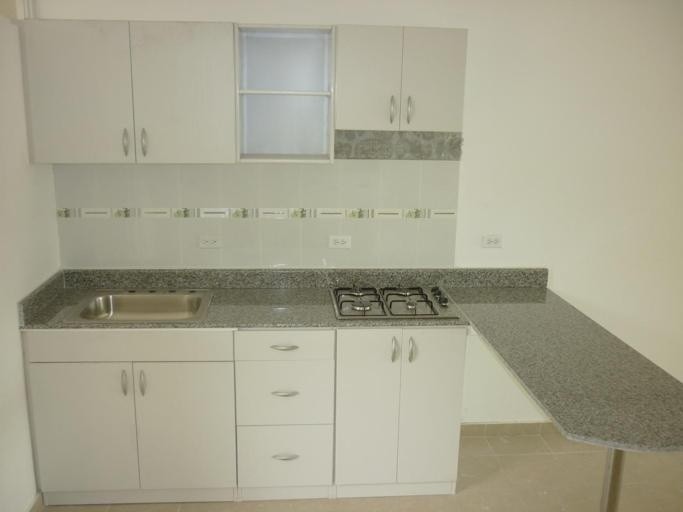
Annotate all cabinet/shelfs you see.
[234,327,336,502]
[234,24,335,163]
[334,24,467,162]
[19,19,236,164]
[334,327,467,498]
[20,328,238,507]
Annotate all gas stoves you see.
[328,283,459,319]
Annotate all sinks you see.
[67,285,214,326]
